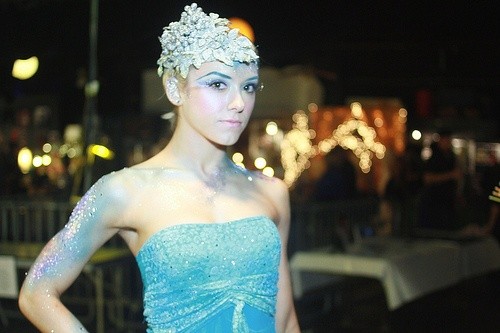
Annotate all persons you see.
[19,3,301,333]
[289,127,500,251]
[0,145,115,246]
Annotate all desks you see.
[1,242,133,333]
[290,235,500,333]
